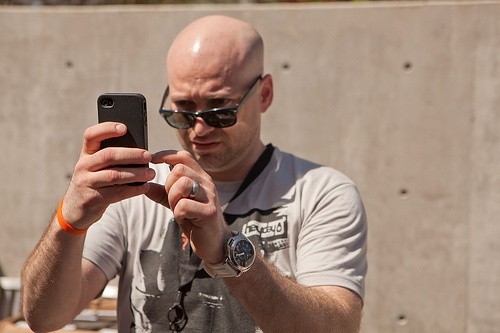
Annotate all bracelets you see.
[56,198,88,235]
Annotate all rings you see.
[190,180,200,198]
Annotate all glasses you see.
[159,74,262,130]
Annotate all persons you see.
[21,14,368,333]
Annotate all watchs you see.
[201,230,256,279]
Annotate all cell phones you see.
[97,93,148,186]
